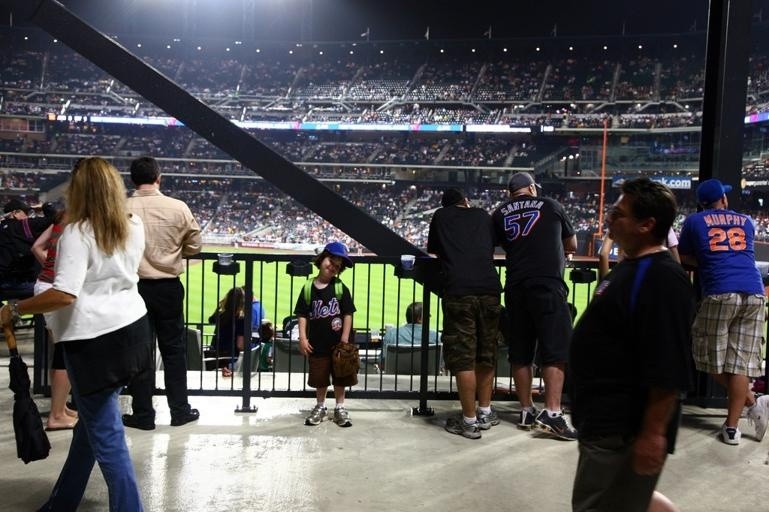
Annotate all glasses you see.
[606,204,625,220]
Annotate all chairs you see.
[270,337,310,372]
[375,342,443,375]
[182,324,207,372]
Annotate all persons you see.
[376,300,445,375]
[2,47,768,257]
[121,156,204,432]
[598,176,683,281]
[425,185,508,442]
[487,172,579,442]
[0,154,155,512]
[494,302,510,353]
[28,209,80,431]
[677,178,769,447]
[294,241,361,429]
[0,198,67,304]
[567,174,694,512]
[205,283,277,374]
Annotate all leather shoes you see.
[121,414,155,430]
[171,409,198,426]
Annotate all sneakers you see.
[532,408,578,440]
[721,423,742,444]
[477,405,500,429]
[517,406,540,431]
[747,395,768,441]
[305,406,328,425]
[445,415,481,438]
[333,407,353,427]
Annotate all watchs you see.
[8,299,24,317]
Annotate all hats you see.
[325,242,353,268]
[3,199,31,215]
[441,186,472,207]
[509,171,543,191]
[42,197,64,218]
[697,178,733,205]
